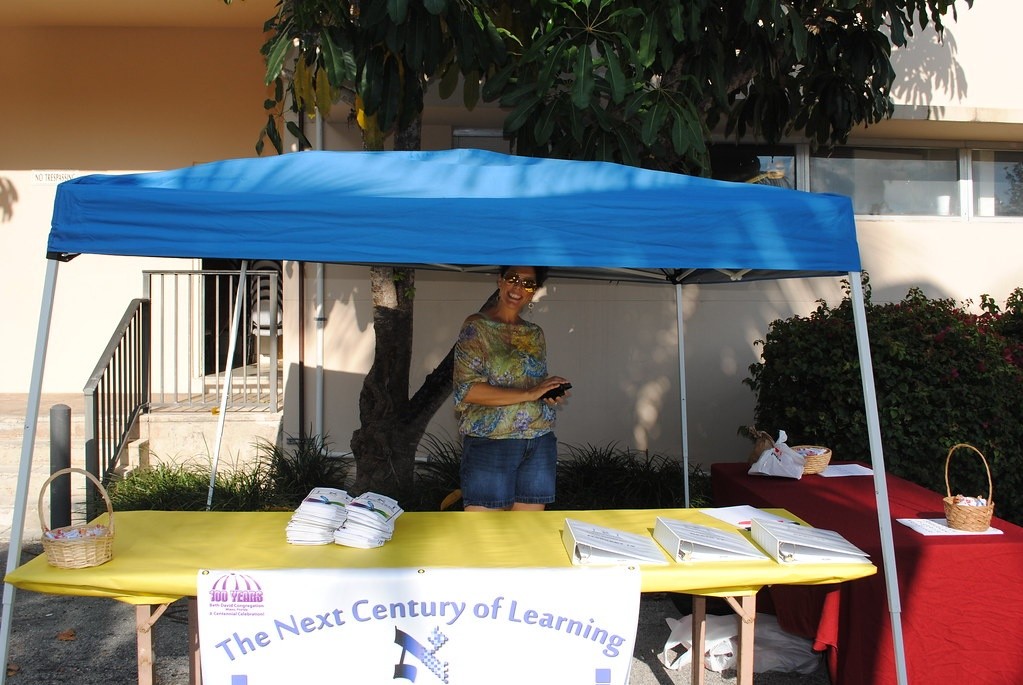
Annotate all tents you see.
[0,147,907,685]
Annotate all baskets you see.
[38,469,116,568]
[942,444,995,531]
[790,445,832,474]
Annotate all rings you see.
[553,402,558,405]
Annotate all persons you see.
[453,266,571,511]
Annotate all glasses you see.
[501,272,537,288]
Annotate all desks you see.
[4,508,877,685]
[710,461,1023,685]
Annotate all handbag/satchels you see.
[748,430,805,480]
[657,613,822,673]
